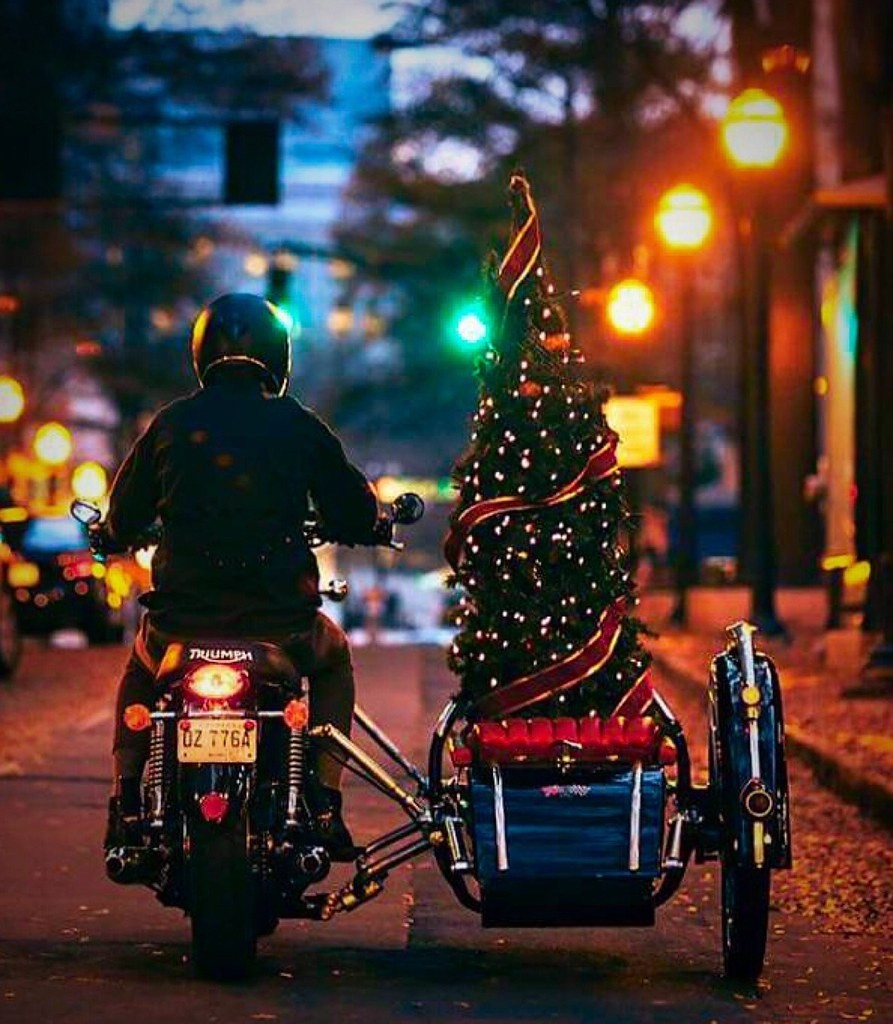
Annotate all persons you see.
[91,295,394,878]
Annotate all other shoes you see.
[103,797,139,850]
[313,788,356,861]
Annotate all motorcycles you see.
[70,491,794,995]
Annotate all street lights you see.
[652,182,715,621]
[726,86,789,640]
[32,420,72,511]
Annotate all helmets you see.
[192,293,290,397]
